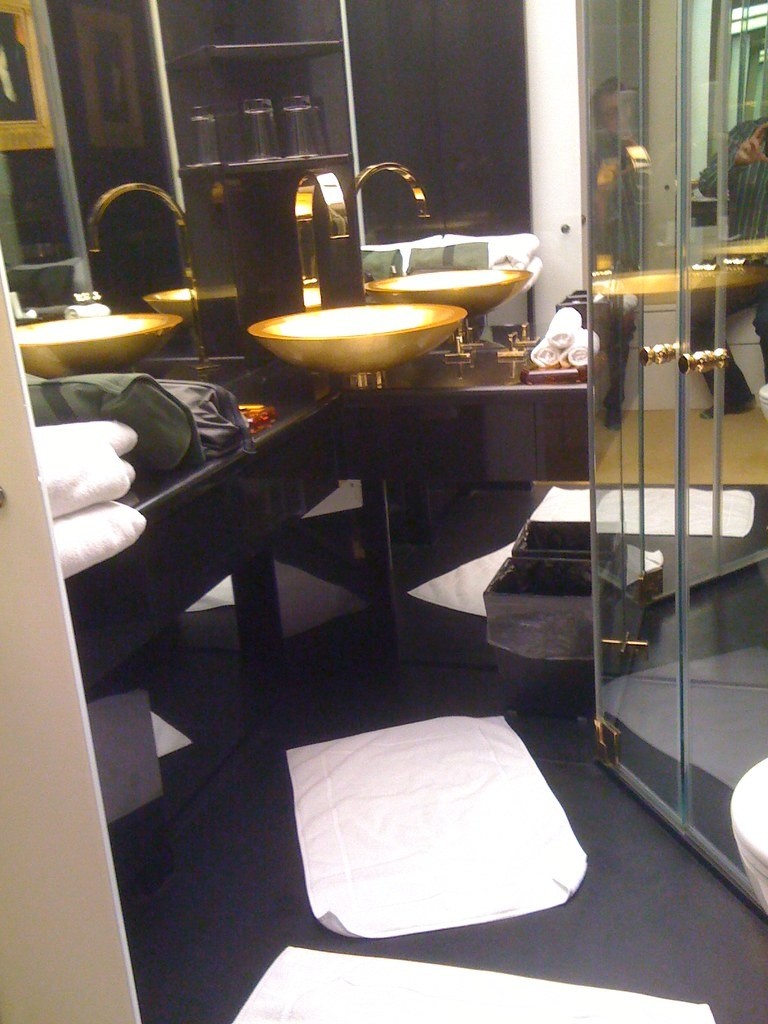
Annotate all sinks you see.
[364,267,533,318]
[16,313,182,379]
[141,287,193,327]
[240,304,468,374]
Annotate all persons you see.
[589,81,652,429]
[689,116,768,419]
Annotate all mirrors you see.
[340,0,588,346]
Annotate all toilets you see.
[728,755,767,915]
[757,384,768,419]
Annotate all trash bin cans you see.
[485,554,617,716]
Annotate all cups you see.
[280,95,319,159]
[188,105,220,165]
[242,98,281,162]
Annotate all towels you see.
[360,231,543,289]
[530,306,600,367]
[23,417,148,581]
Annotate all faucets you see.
[85,182,196,307]
[354,163,430,220]
[293,168,351,311]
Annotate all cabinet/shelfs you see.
[172,36,351,175]
